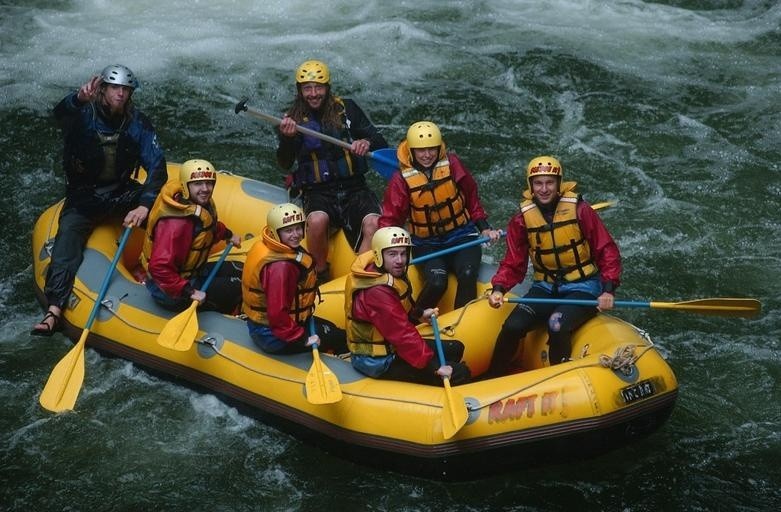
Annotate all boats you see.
[34,156,681,460]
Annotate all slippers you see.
[30,309,65,338]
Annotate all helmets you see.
[369,224,418,269]
[177,157,217,201]
[96,63,139,96]
[265,201,310,243]
[525,154,566,203]
[404,119,444,164]
[294,58,332,87]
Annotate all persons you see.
[473,156,621,382]
[242,203,350,355]
[139,158,243,316]
[31,64,166,336]
[377,122,502,324]
[344,227,471,386]
[276,59,388,284]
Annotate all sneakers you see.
[316,261,331,284]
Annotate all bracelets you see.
[492,284,506,296]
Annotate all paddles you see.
[156,241,233,353]
[502,297,762,317]
[431,313,469,440]
[406,200,613,267]
[233,98,402,185]
[304,312,342,406]
[40,219,136,410]
